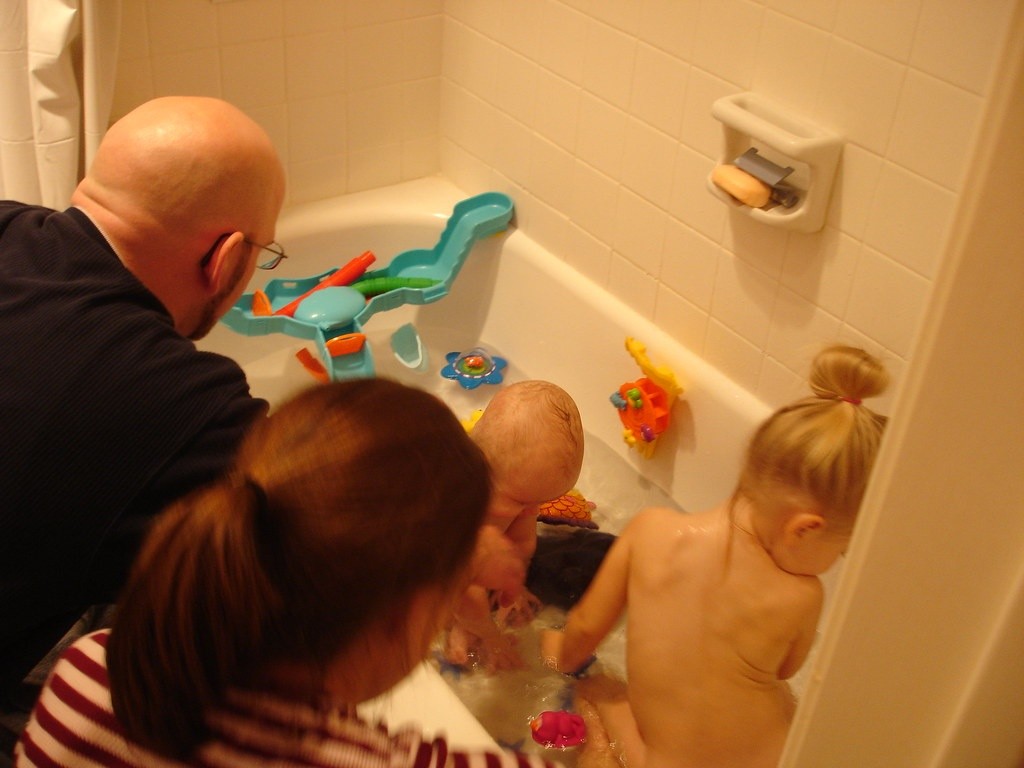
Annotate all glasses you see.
[200,233,289,270]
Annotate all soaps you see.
[710,163,774,208]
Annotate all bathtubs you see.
[186,175,848,753]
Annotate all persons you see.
[431,379,585,675]
[543,344,888,768]
[0,97,270,767]
[7,378,574,768]
